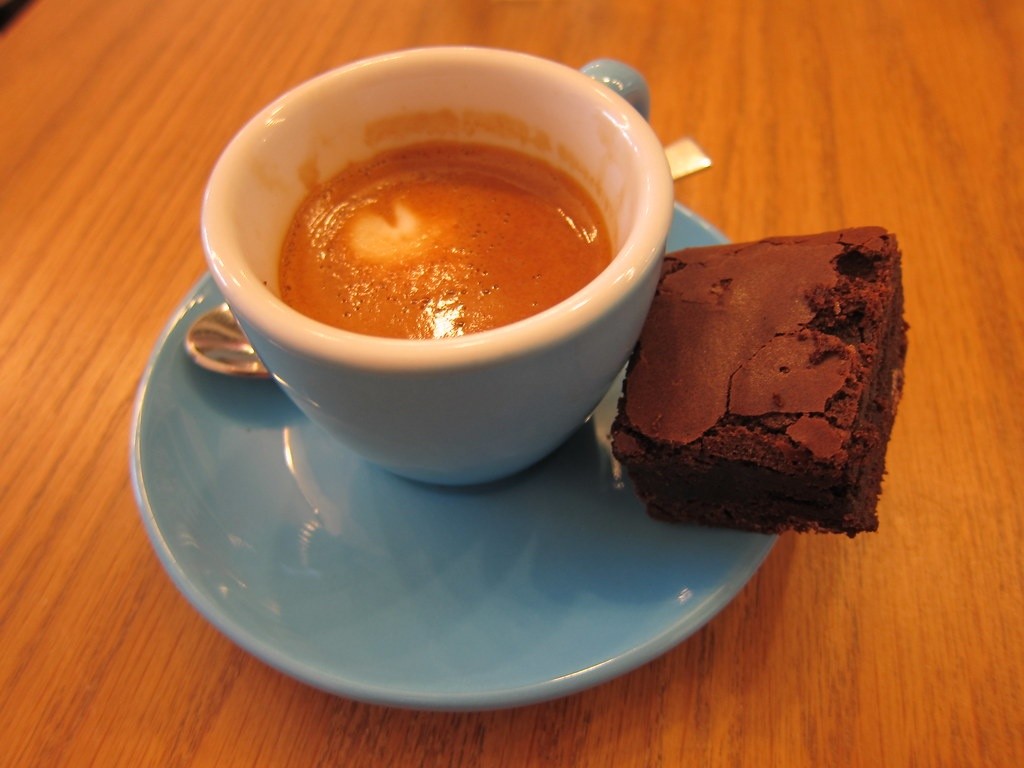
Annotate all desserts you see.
[609,226,911,536]
